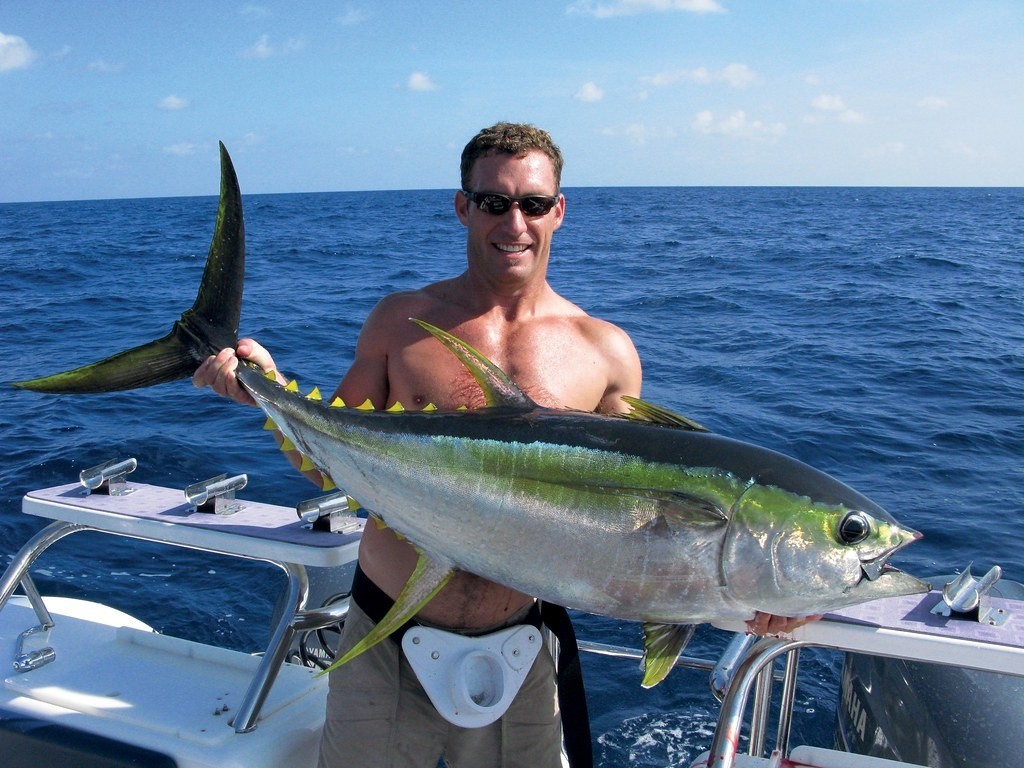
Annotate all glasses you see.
[460,186,560,218]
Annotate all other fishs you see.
[11,141,932,729]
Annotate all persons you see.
[193,120,823,768]
